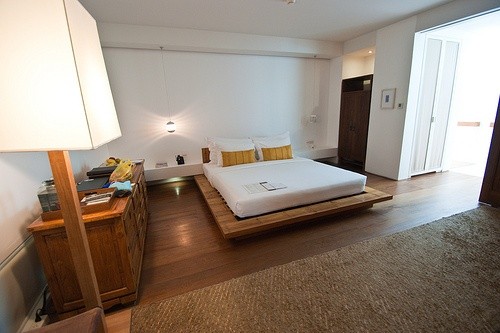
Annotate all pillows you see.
[253,130,295,161]
[221,149,255,167]
[261,143,293,160]
[215,138,258,167]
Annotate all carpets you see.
[129,204,500,333]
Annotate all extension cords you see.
[30,314,50,329]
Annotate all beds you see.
[194,154,394,241]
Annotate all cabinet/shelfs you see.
[410,34,461,181]
[27,159,151,322]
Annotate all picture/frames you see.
[379,88,397,110]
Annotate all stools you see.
[18,307,105,333]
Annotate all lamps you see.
[0,0,123,332]
[165,120,177,134]
